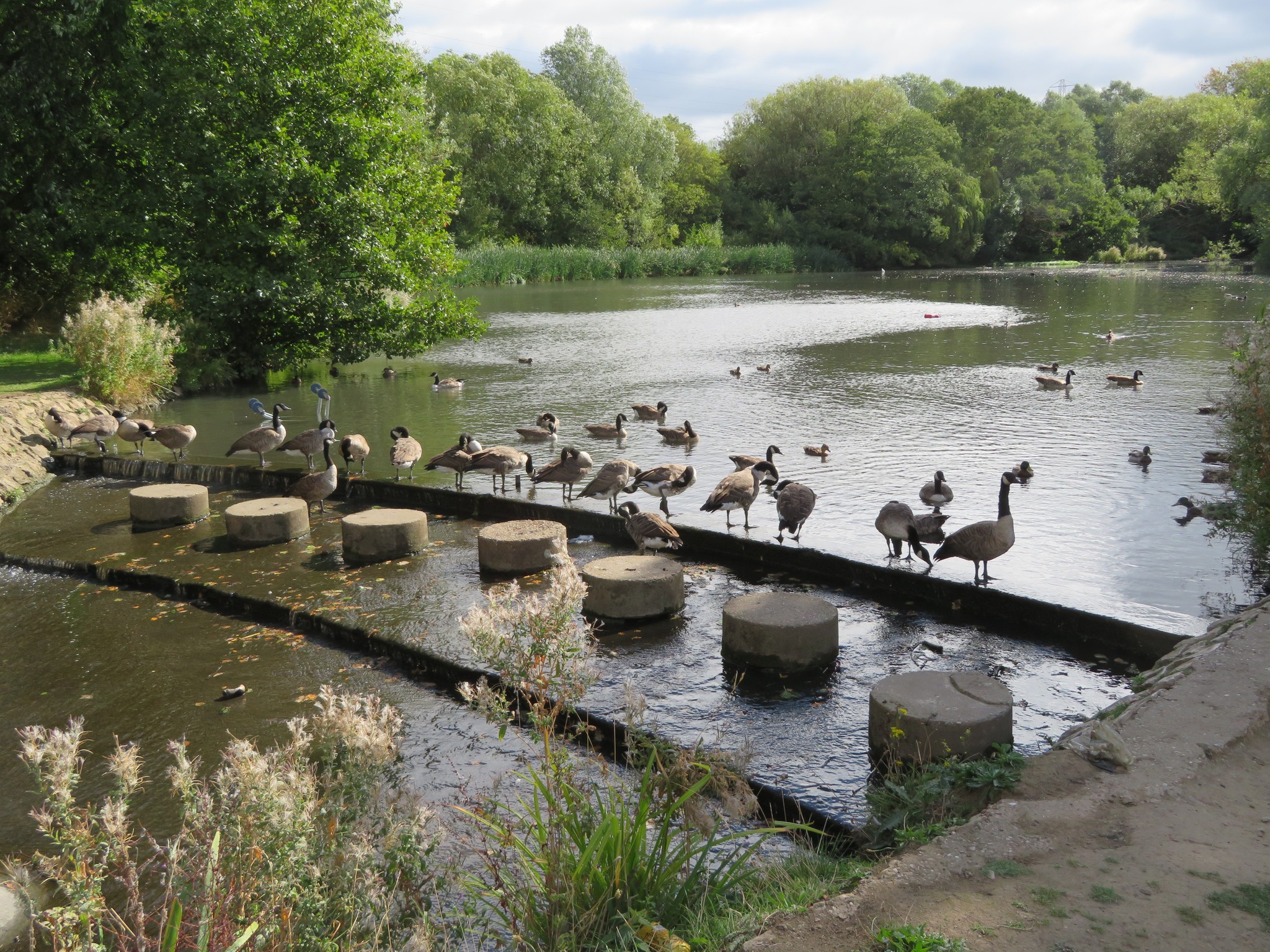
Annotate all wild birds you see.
[225,403,816,558]
[1198,401,1225,413]
[517,357,534,363]
[1128,446,1153,464]
[734,303,741,307]
[45,407,198,460]
[1107,369,1145,384]
[1199,450,1231,461]
[757,364,771,371]
[804,444,832,457]
[428,372,468,388]
[1203,469,1236,480]
[1035,369,1077,388]
[381,368,397,377]
[1172,497,1237,517]
[1221,285,1248,300]
[1038,363,1061,372]
[919,471,954,502]
[1106,329,1114,338]
[730,366,741,375]
[932,471,1024,585]
[1014,461,1034,477]
[876,499,952,568]
